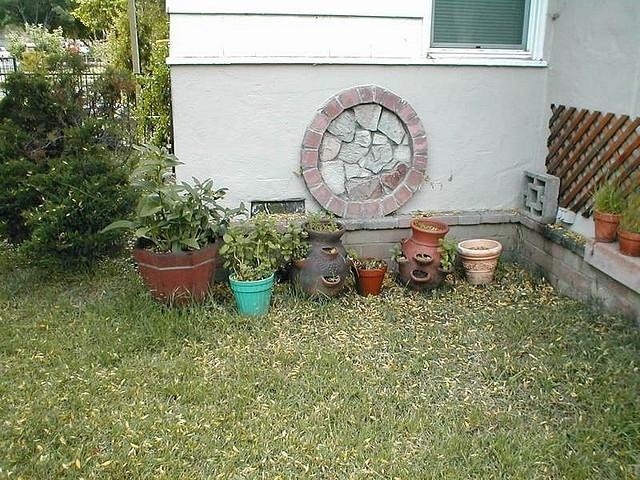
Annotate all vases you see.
[456,238,503,286]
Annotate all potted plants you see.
[348,249,388,299]
[218,209,310,316]
[93,141,250,311]
[591,175,624,243]
[288,207,352,301]
[388,217,463,292]
[615,191,640,257]
